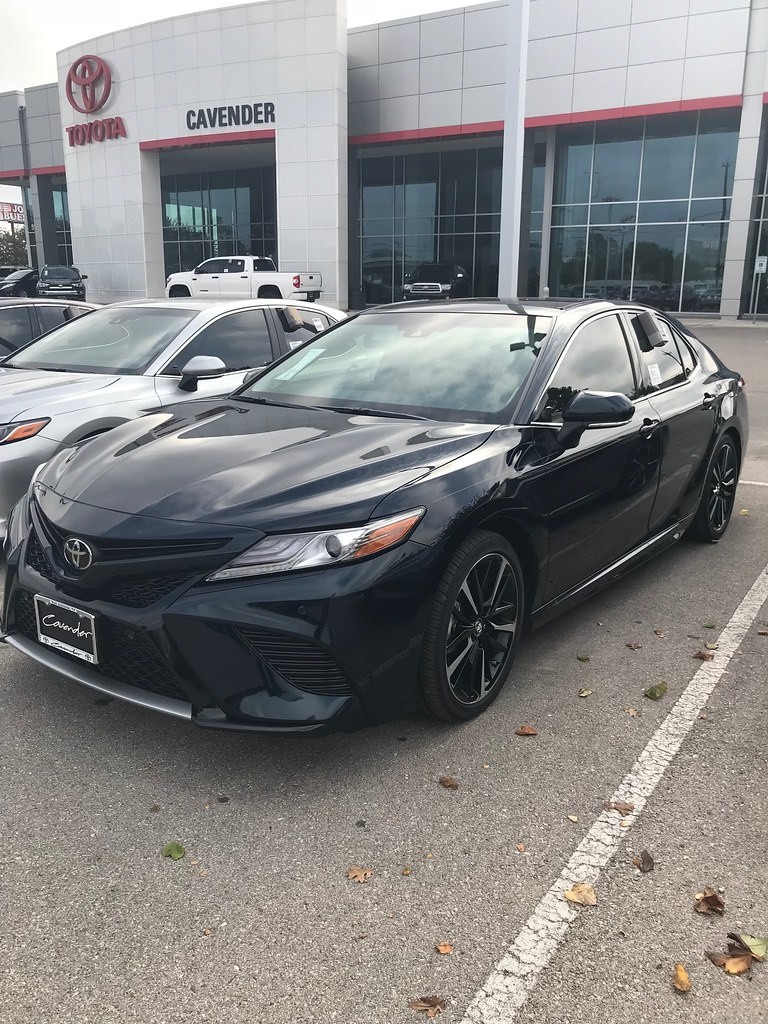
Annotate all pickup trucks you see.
[164,255,324,302]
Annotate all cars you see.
[34,262,88,302]
[556,277,722,311]
[0,296,146,369]
[0,296,384,542]
[1,300,749,742]
[0,265,34,282]
[0,270,40,299]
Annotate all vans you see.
[404,261,469,305]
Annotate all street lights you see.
[618,214,636,284]
[601,196,618,303]
[712,157,733,287]
